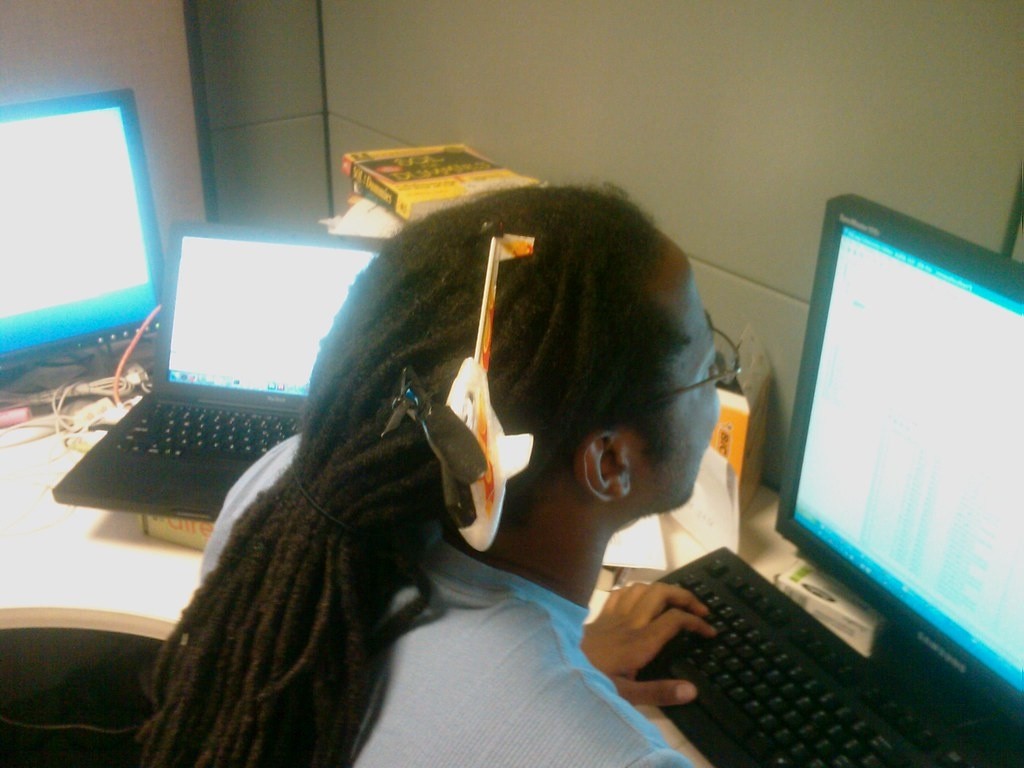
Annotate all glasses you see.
[632,327,742,409]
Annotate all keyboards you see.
[634,547,997,768]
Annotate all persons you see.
[132,184,721,767]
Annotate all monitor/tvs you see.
[0,87,167,385]
[775,192,1024,768]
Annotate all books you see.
[342,142,546,225]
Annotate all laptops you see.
[53,215,385,521]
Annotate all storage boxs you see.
[774,557,886,654]
[708,324,773,516]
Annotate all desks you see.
[0,333,876,768]
